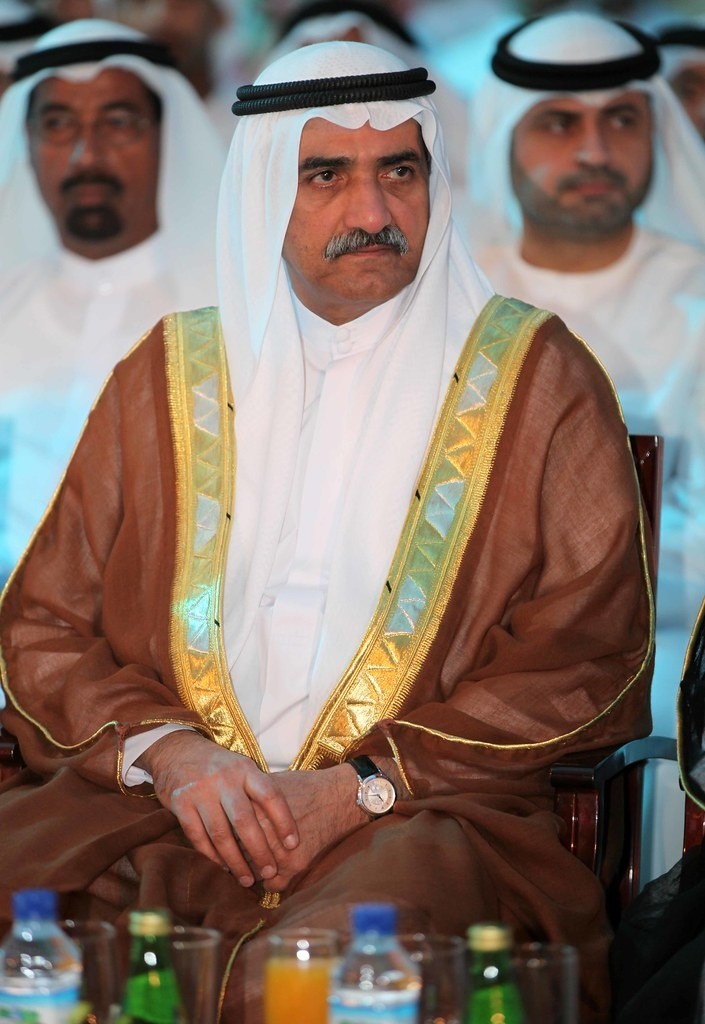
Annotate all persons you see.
[0,18,227,591]
[449,10,705,897]
[0,41,657,1024]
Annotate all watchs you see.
[346,754,396,822]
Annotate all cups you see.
[169,926,224,1024]
[509,943,579,1024]
[262,927,339,1024]
[57,918,118,1024]
[396,934,469,1024]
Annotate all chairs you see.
[548,432,680,929]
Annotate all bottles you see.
[114,910,186,1024]
[464,921,526,1024]
[0,888,83,1024]
[328,901,421,1024]
